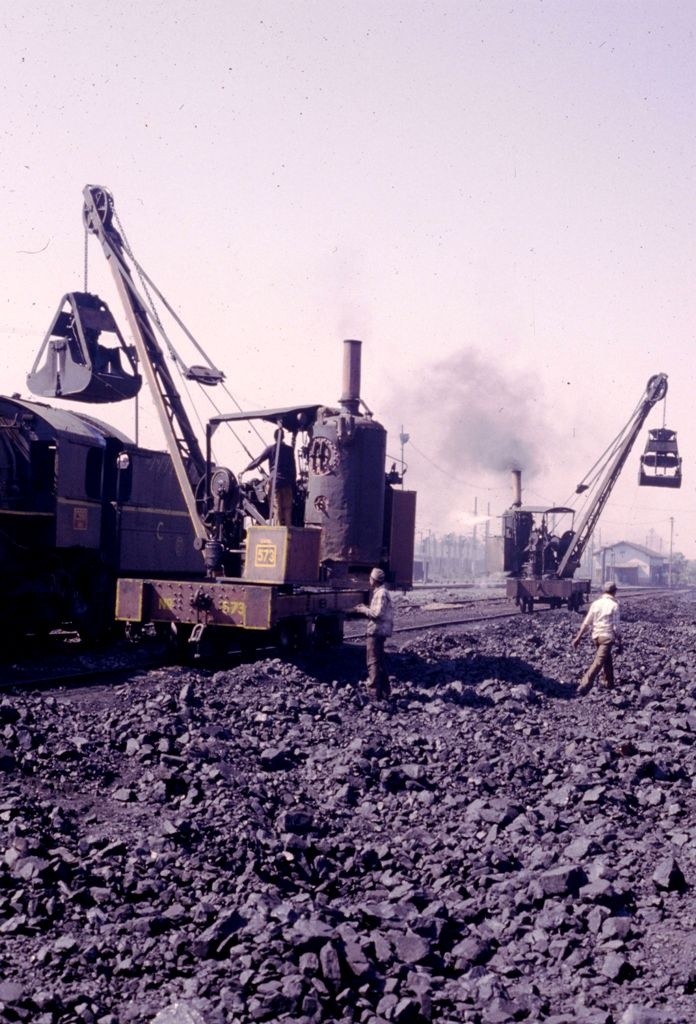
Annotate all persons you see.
[242,428,297,526]
[356,568,393,688]
[571,582,623,697]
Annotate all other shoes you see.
[380,690,390,699]
[364,686,379,696]
[603,679,614,688]
[576,685,587,696]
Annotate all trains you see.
[0,392,242,671]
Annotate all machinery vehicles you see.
[25,182,417,667]
[506,363,683,612]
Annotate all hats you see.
[369,568,385,582]
[604,581,616,592]
